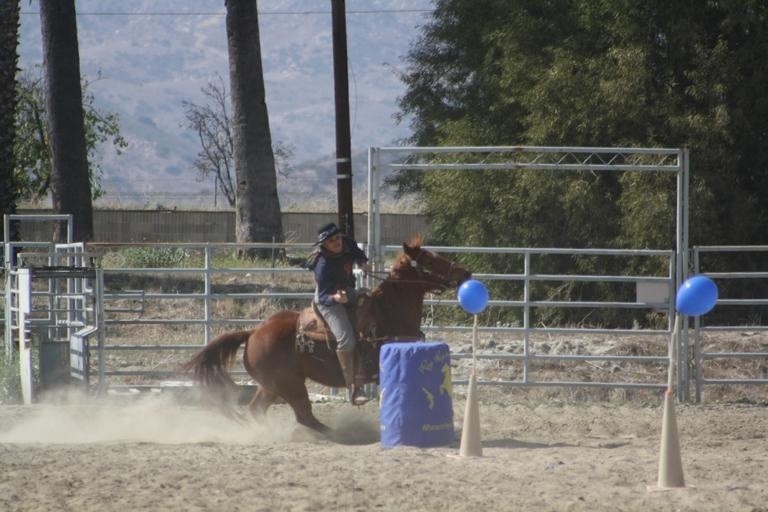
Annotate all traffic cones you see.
[632,385,699,494]
[445,373,492,461]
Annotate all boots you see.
[336,350,370,403]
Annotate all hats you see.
[312,223,344,247]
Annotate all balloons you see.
[456,280,489,315]
[674,275,718,319]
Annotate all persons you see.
[310,222,371,405]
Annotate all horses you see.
[179,234,472,437]
